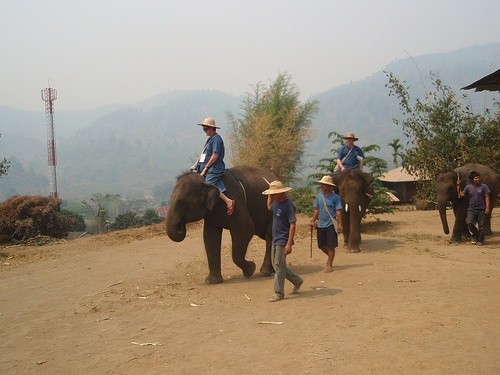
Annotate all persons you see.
[334,132,372,198]
[262,181,303,301]
[310,176,342,272]
[190,118,234,215]
[456,171,490,245]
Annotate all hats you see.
[316,176,337,187]
[342,132,359,141]
[261,180,292,195]
[197,117,221,129]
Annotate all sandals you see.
[226,200,235,216]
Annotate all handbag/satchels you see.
[333,145,355,175]
[332,219,339,233]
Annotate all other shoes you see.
[292,277,304,294]
[477,242,483,246]
[471,240,476,244]
[268,292,284,302]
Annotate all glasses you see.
[203,128,209,132]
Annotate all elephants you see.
[166,164,284,286]
[437,163,498,245]
[331,168,373,253]
